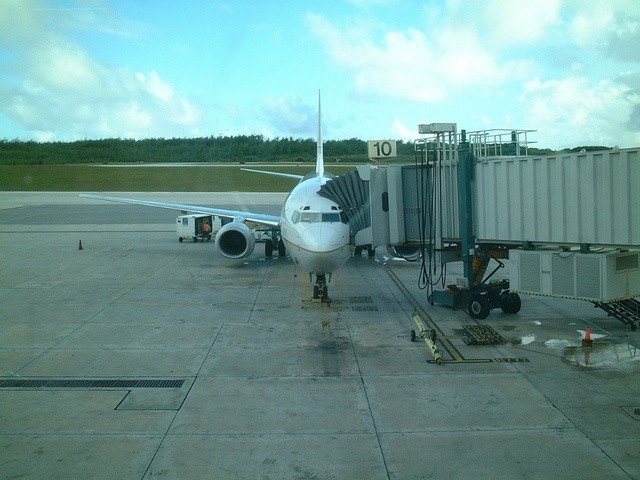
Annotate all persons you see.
[201,222,211,242]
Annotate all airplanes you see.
[78,90,375,300]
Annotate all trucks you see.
[176,214,221,242]
